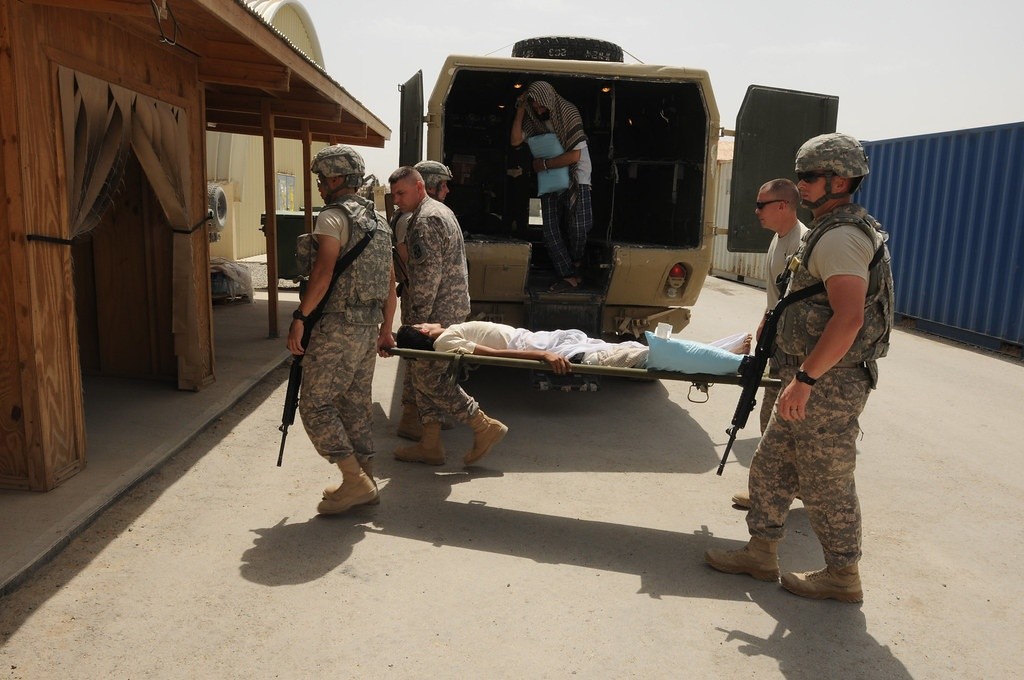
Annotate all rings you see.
[791,408,797,411]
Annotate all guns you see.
[276,278,320,468]
[715,269,793,478]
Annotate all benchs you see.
[587,235,697,299]
[465,234,533,302]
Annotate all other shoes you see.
[547,280,584,294]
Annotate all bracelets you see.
[543,159,547,170]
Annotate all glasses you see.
[797,171,838,183]
[757,200,789,209]
[316,177,327,184]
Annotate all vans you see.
[399,36,839,343]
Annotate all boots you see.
[463,409,508,466]
[733,490,752,508]
[323,463,381,505]
[317,454,377,514]
[397,407,424,441]
[706,538,780,581]
[441,420,455,429]
[393,423,446,465]
[781,562,863,603]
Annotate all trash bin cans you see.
[259,210,331,279]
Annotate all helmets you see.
[310,145,365,176]
[795,133,870,178]
[413,161,452,189]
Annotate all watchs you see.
[796,369,816,386]
[293,309,307,322]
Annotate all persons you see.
[286,145,397,514]
[387,166,508,465]
[397,320,756,377]
[389,161,453,441]
[511,80,593,294]
[704,132,894,601]
[732,178,809,508]
